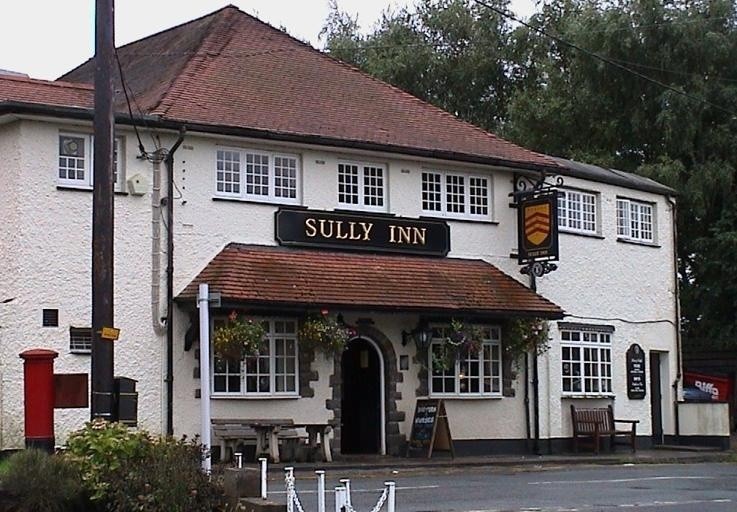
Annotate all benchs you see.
[571,404,640,456]
[209,417,299,462]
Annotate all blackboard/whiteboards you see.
[409,399,441,448]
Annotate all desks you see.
[250,424,343,463]
